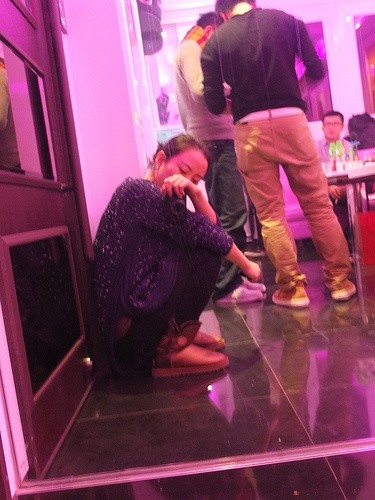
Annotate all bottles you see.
[329,141,358,172]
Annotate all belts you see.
[239,106,303,125]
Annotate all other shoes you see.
[349,252,362,266]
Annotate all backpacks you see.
[348,112,375,149]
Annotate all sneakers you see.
[216,276,267,304]
[332,277,356,302]
[272,274,310,308]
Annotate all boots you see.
[151,318,229,378]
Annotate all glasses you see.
[324,122,342,127]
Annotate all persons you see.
[176,13,266,305]
[92,133,262,378]
[316,110,354,263]
[201,0,356,308]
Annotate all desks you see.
[316,149,375,326]
